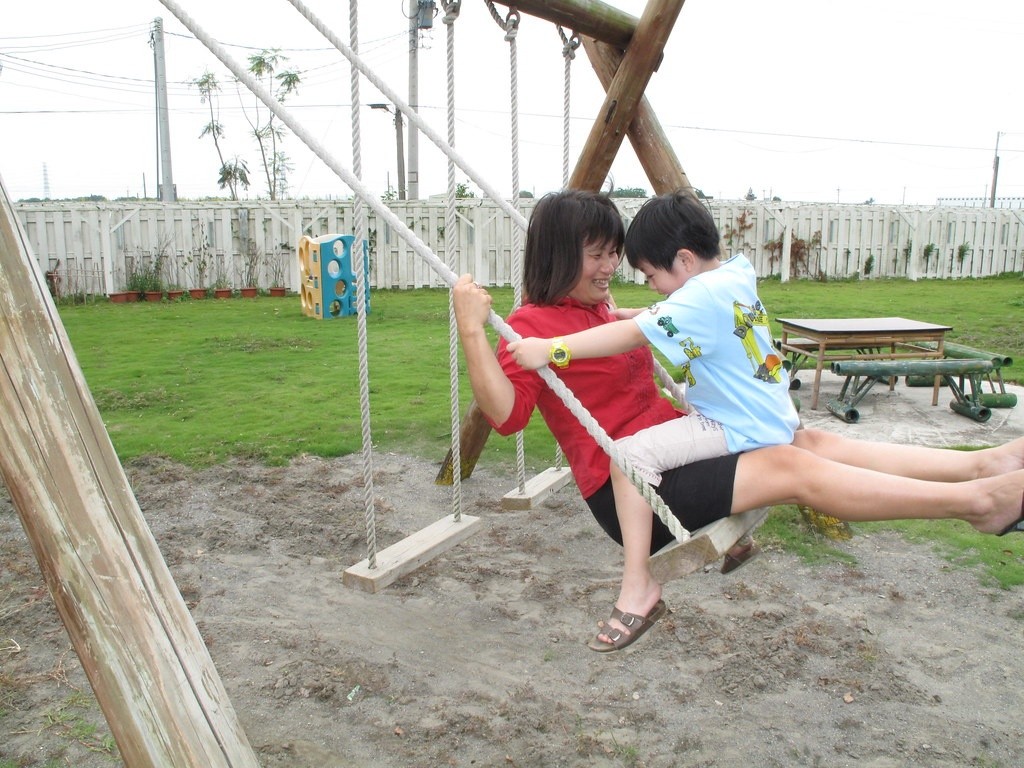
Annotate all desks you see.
[775,317,954,410]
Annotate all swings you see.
[339,0,486,595]
[498,9,583,513]
[162,0,773,586]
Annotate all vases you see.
[108,291,128,303]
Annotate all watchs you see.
[549,336,571,369]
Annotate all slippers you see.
[587,598,677,654]
[995,492,1024,537]
[720,539,764,576]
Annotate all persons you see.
[507,187,801,653]
[453,192,1024,556]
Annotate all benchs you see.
[825,357,994,424]
[773,337,898,390]
[772,346,801,412]
[905,341,1017,408]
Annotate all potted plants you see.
[211,251,231,299]
[180,213,214,300]
[263,241,297,297]
[231,222,264,298]
[125,270,143,302]
[141,223,176,301]
[163,244,183,300]
